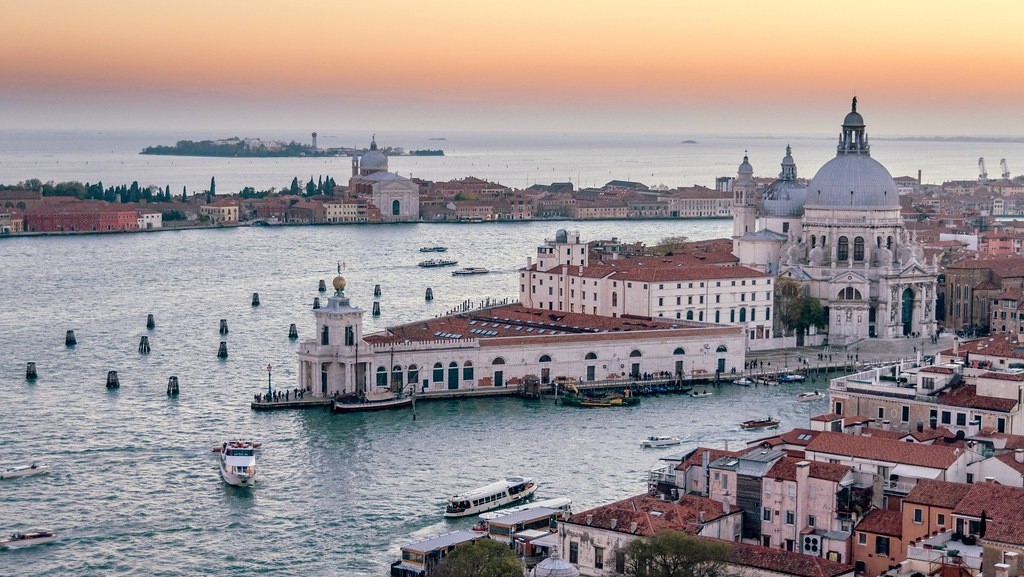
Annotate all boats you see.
[212,437,262,487]
[691,393,712,397]
[559,382,642,407]
[443,476,538,518]
[776,374,803,381]
[451,268,489,275]
[418,258,459,267]
[489,507,570,548]
[419,246,448,252]
[641,435,681,446]
[795,390,825,402]
[732,378,750,386]
[740,417,781,430]
[752,376,779,386]
[619,385,692,395]
[470,497,572,533]
[389,529,492,577]
[0,465,50,481]
[1,530,60,548]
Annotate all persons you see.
[818,353,832,360]
[505,380,508,387]
[745,358,771,369]
[410,387,413,396]
[846,352,858,360]
[797,355,809,365]
[253,388,305,401]
[628,371,668,380]
[936,328,940,337]
[907,332,920,338]
[420,385,425,393]
[913,346,916,351]
[932,337,938,344]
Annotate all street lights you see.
[267,363,273,401]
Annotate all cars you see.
[340,391,356,400]
